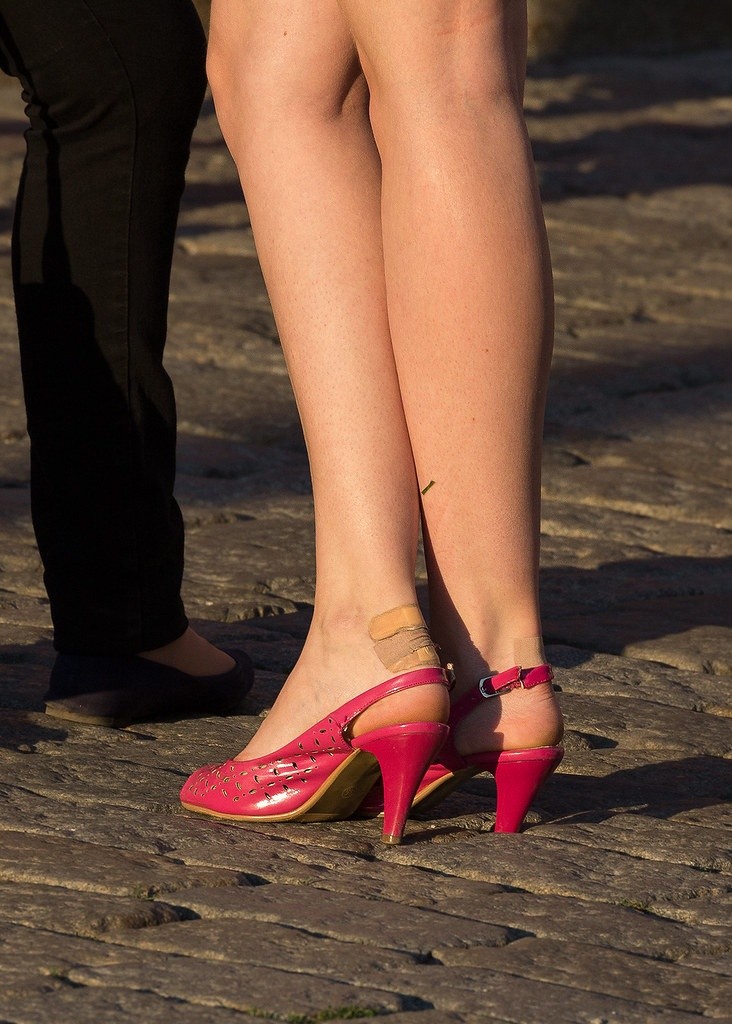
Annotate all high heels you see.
[353,663,565,833]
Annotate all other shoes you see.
[180,668,457,845]
[45,652,255,728]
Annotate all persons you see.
[0,0,255,725]
[179,0,569,846]
